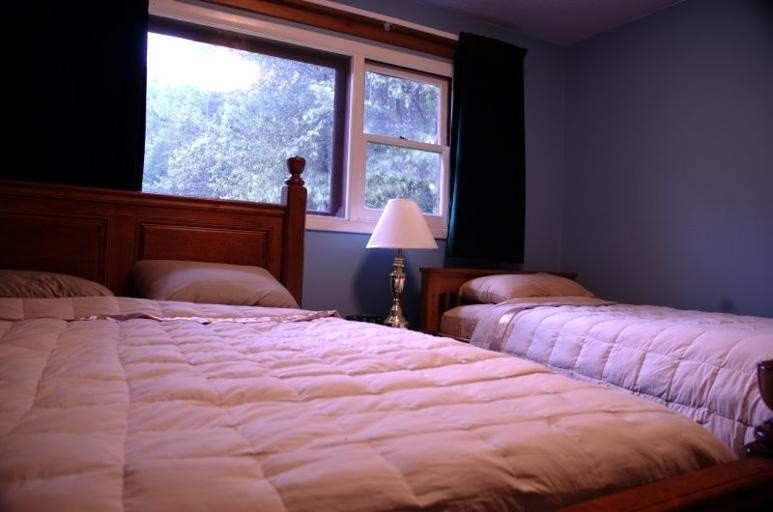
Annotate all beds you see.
[457,269,598,306]
[417,267,773,455]
[0,156,772,510]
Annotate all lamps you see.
[364,199,439,327]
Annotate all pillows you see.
[136,259,301,309]
[0,269,115,299]
